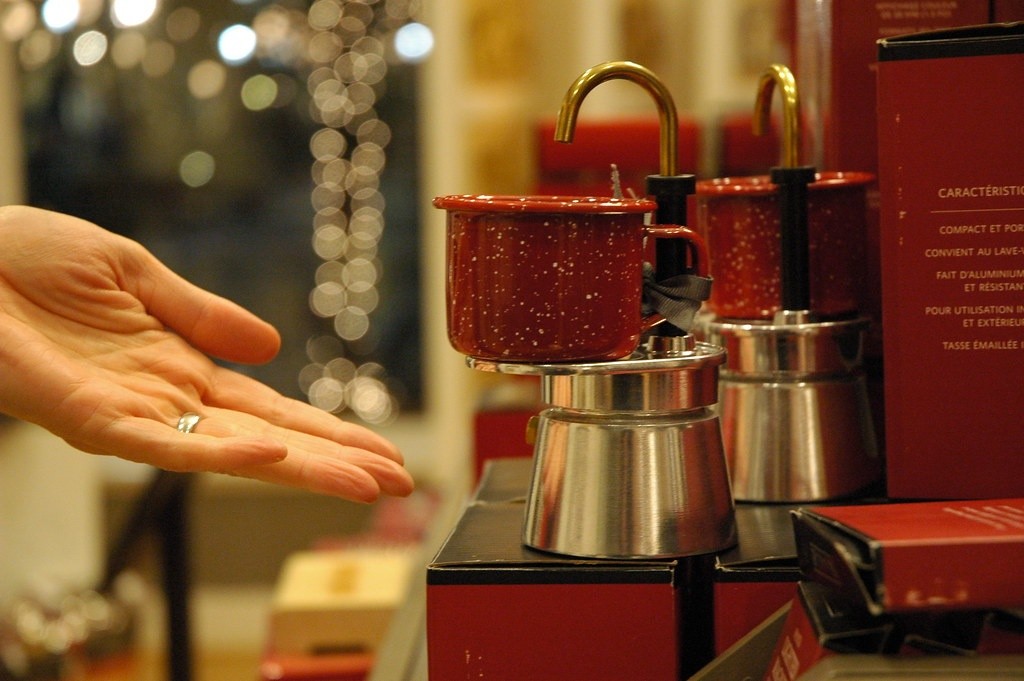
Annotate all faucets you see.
[554,59,679,177]
[751,62,802,168]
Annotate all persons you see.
[0,205,413,503]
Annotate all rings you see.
[177,412,203,433]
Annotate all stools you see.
[268,545,421,655]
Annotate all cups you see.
[695,172,877,320]
[434,194,708,364]
